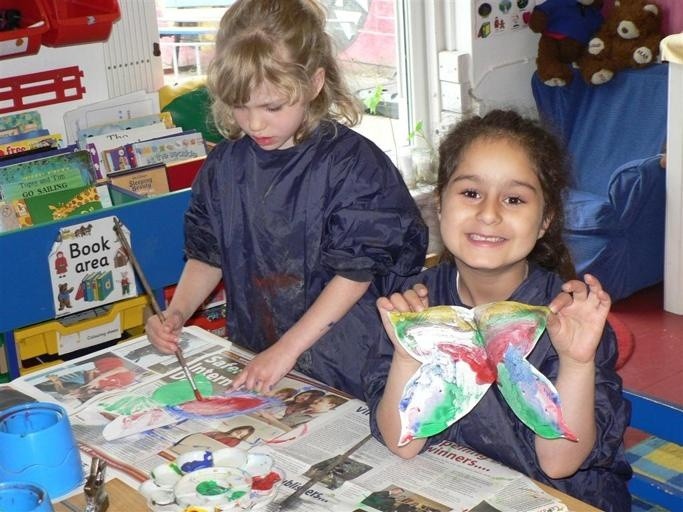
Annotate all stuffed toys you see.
[526,0,603,86]
[575,1,663,87]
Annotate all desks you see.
[0,326,604,512]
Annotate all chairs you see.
[530,62,668,304]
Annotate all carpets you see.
[622,389,683,511]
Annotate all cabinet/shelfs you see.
[2,142,228,383]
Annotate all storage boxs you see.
[0,0,120,61]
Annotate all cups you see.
[0,480,50,511]
[0,401,84,499]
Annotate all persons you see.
[141,0,429,402]
[362,488,446,510]
[200,425,253,448]
[265,381,347,424]
[362,110,632,511]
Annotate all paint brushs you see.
[113,217,203,403]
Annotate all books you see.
[0,88,206,231]
